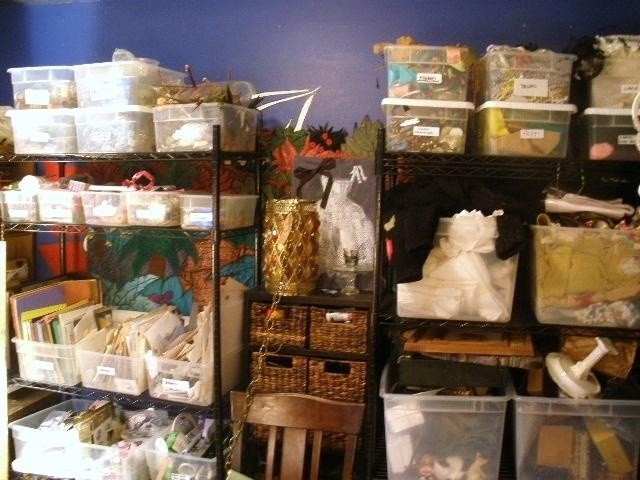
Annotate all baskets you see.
[247,300,373,452]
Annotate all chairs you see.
[226,389,367,480]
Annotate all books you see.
[10,277,113,386]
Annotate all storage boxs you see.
[376,33,640,154]
[378,346,640,478]
[3,382,227,480]
[379,180,640,346]
[2,330,246,405]
[1,56,257,231]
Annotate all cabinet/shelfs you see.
[238,288,372,479]
